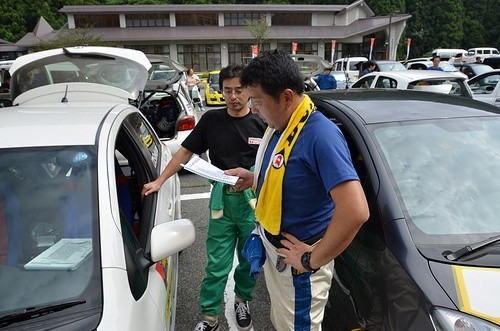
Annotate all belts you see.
[263,228,327,249]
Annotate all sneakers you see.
[192,320,221,331]
[234,301,252,331]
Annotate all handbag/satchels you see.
[192,86,202,105]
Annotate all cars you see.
[0,54,201,165]
[0,45,195,331]
[203,47,500,107]
[302,89,500,331]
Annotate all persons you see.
[141,63,267,331]
[426,56,444,72]
[225,50,370,331]
[359,61,375,89]
[184,68,206,111]
[317,66,337,90]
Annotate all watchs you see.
[301,251,320,273]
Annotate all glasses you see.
[223,89,247,96]
